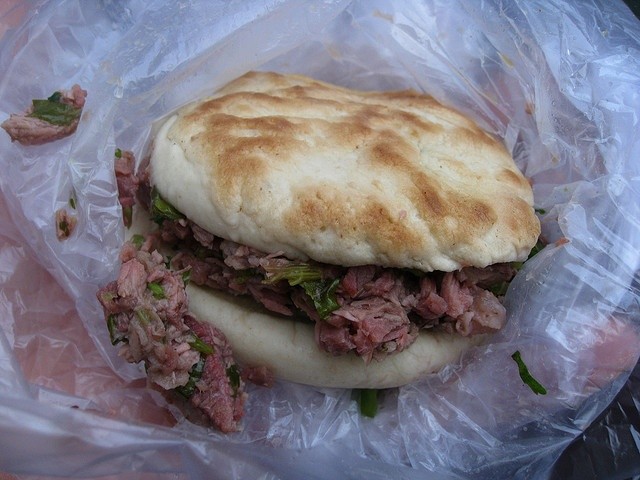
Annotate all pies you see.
[124,71,543,390]
